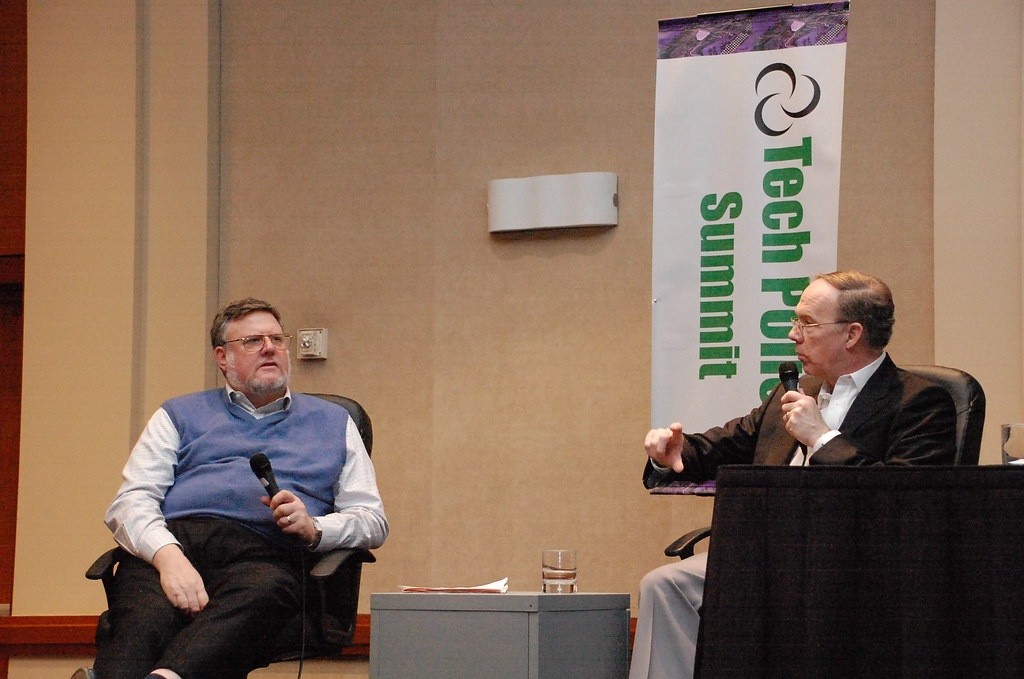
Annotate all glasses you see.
[791,317,848,335]
[224,332,294,352]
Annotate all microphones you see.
[779,361,807,456]
[249,453,304,543]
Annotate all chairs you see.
[664,364,986,559]
[85,393,373,663]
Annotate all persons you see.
[626,271,959,679]
[71,298,388,679]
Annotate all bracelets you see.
[308,518,322,550]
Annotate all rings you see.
[786,412,790,420]
[287,515,292,524]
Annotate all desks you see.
[369,590,630,679]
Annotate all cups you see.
[1000,422,1024,465]
[541,548,578,594]
